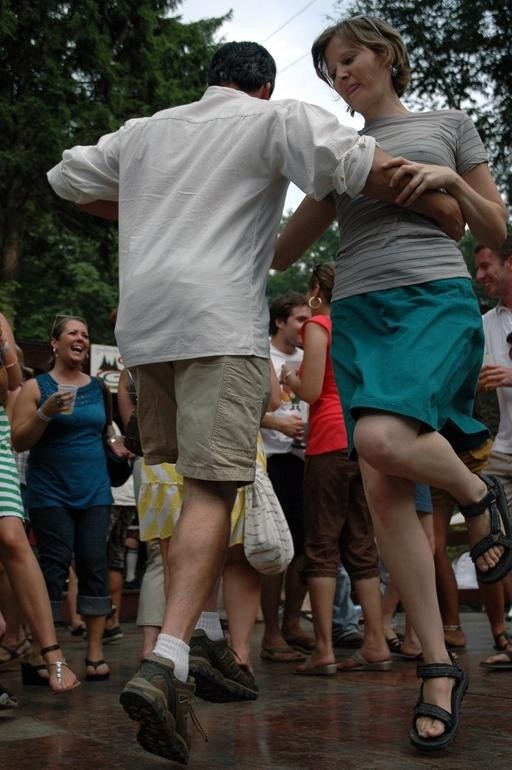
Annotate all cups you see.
[56,384,77,414]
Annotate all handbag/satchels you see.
[241,464,294,576]
[103,439,133,486]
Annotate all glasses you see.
[51,313,70,336]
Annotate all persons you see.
[0,218,512,719]
[44,41,467,764]
[273,12,508,756]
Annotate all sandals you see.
[260,605,512,676]
[408,654,469,753]
[457,473,511,584]
[0,577,141,719]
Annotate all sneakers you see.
[188,629,260,703]
[121,651,198,766]
